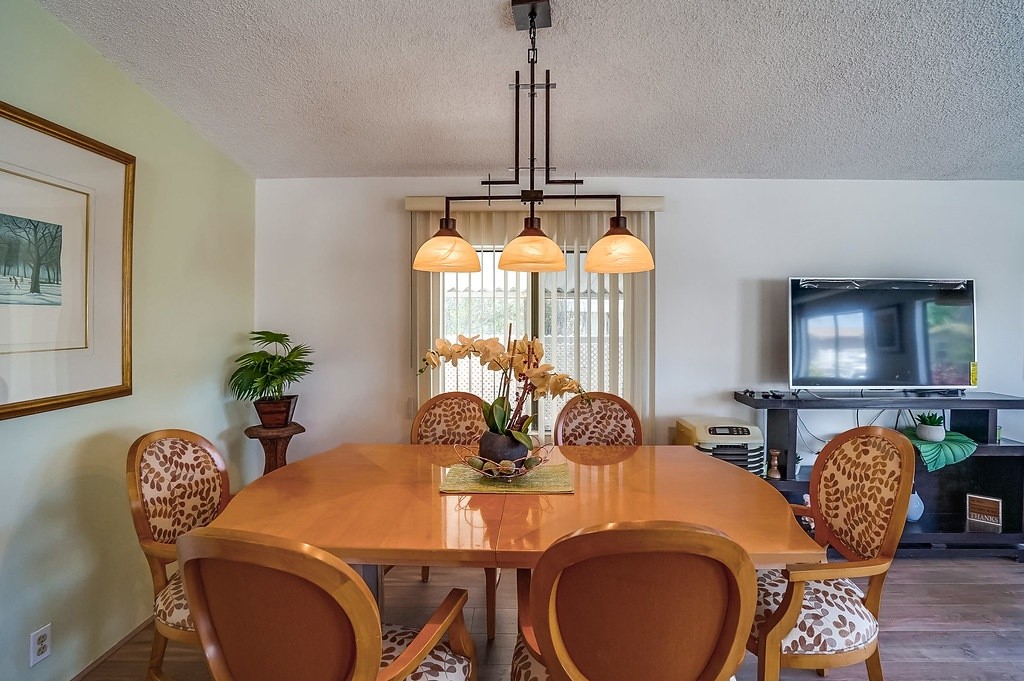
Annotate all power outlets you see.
[30,622,52,667]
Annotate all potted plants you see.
[228,330,315,428]
[915,411,945,442]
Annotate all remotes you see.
[770,390,785,397]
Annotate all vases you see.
[479,430,529,476]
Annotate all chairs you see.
[510,520,757,681]
[126,429,236,681]
[410,392,501,582]
[176,526,477,681]
[554,392,642,446]
[745,426,915,681]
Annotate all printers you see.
[676,415,767,479]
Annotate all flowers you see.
[416,323,593,450]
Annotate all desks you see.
[207,442,825,617]
[244,422,306,476]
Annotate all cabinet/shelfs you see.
[734,390,1024,562]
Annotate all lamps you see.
[412,0,654,273]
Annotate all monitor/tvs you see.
[788,275,978,396]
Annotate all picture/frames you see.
[0,101,136,420]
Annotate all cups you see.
[997,425,1002,443]
